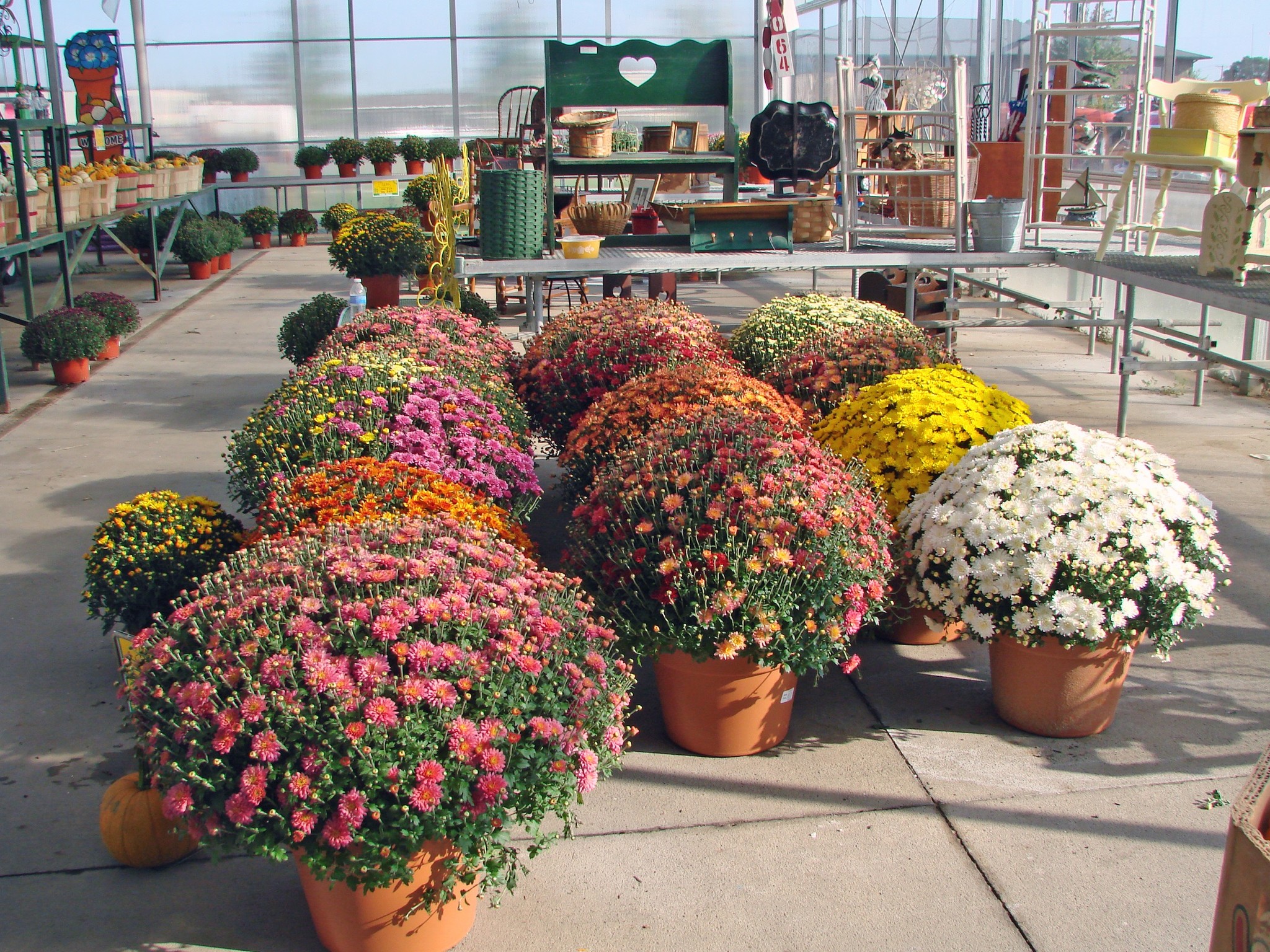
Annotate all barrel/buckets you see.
[968,195,1028,253]
[0,165,204,249]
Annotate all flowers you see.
[20,211,1233,952]
[709,132,759,166]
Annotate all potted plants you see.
[119,135,527,278]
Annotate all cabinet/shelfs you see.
[0,34,219,414]
[546,36,740,250]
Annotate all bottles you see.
[349,279,367,320]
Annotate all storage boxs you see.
[1148,127,1231,158]
[0,163,206,248]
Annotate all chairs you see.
[1094,78,1270,262]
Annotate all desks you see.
[225,177,1270,436]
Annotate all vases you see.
[361,275,399,308]
[290,833,479,952]
[51,354,90,384]
[888,594,966,645]
[739,166,771,184]
[91,334,120,360]
[653,639,797,756]
[991,625,1153,737]
[203,172,217,183]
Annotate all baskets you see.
[567,175,632,235]
[569,122,614,157]
[793,200,836,243]
[476,138,546,261]
[885,123,981,227]
[558,111,617,127]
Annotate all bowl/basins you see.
[556,235,605,259]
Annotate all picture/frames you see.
[625,173,662,207]
[668,120,701,154]
